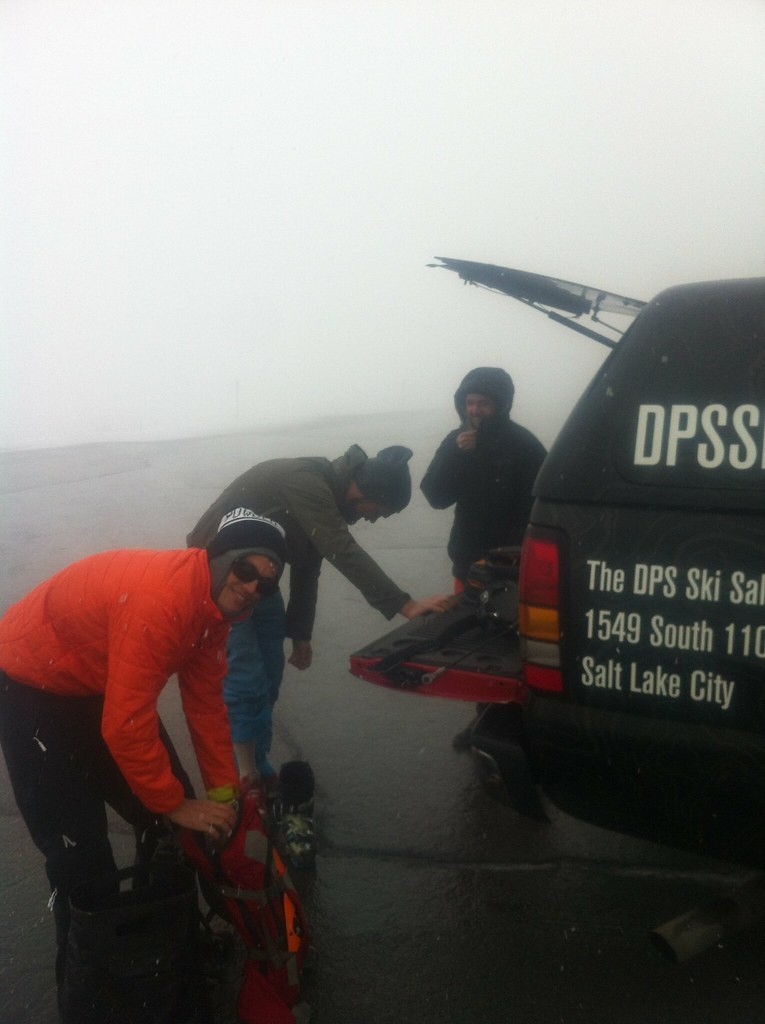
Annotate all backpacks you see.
[179,794,310,1024]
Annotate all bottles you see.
[208,788,238,850]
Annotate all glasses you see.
[232,556,280,597]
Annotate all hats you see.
[355,446,413,512]
[206,506,286,577]
[465,378,499,410]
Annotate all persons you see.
[0,508,286,989]
[186,444,460,804]
[419,366,551,597]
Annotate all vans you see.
[352,249,764,879]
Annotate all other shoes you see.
[453,715,476,746]
